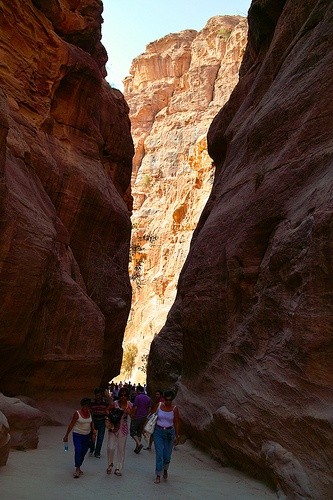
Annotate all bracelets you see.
[91,429,97,434]
[176,434,181,438]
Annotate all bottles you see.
[173,432,181,446]
[64,440,69,454]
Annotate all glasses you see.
[120,394,127,397]
[165,398,172,401]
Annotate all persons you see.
[153,390,181,484]
[104,387,135,476]
[88,389,108,459]
[101,380,165,454]
[63,396,96,479]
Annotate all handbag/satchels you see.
[105,403,124,432]
[144,402,162,434]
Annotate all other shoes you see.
[106,463,113,474]
[94,453,100,458]
[89,449,94,456]
[154,478,160,483]
[134,443,143,454]
[114,468,121,476]
[163,472,168,481]
[143,447,151,451]
[73,470,83,478]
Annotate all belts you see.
[156,424,173,430]
